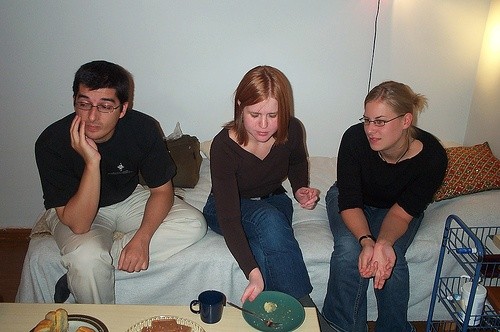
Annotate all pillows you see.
[432,142,500,202]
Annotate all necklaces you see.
[378,137,409,165]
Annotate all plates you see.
[127,315,205,332]
[242,291,305,332]
[30,315,108,332]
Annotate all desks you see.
[0,302,320,332]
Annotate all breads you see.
[142,319,192,332]
[75,326,95,332]
[264,302,278,313]
[33,308,69,332]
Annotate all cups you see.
[190,290,226,324]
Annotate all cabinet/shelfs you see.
[424,214,500,332]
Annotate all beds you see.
[16,147,500,322]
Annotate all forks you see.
[227,302,282,329]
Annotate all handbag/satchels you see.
[136,134,203,188]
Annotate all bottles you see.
[457,282,487,326]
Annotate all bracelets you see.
[359,235,376,243]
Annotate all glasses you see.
[74,101,122,113]
[358,114,406,128]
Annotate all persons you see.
[202,66,330,332]
[320,81,447,332]
[35,60,176,304]
